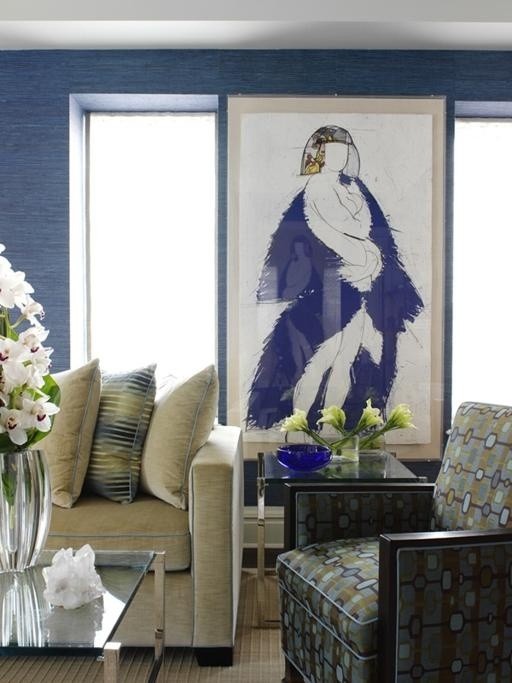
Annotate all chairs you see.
[281,401,511,681]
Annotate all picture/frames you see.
[226,93,447,461]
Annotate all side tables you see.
[253,451,427,628]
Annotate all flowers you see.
[0,243,59,506]
[283,403,413,452]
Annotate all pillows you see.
[44,362,218,510]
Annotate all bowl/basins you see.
[276,443,334,473]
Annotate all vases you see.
[311,436,386,464]
[1,450,55,567]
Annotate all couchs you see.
[1,422,241,667]
